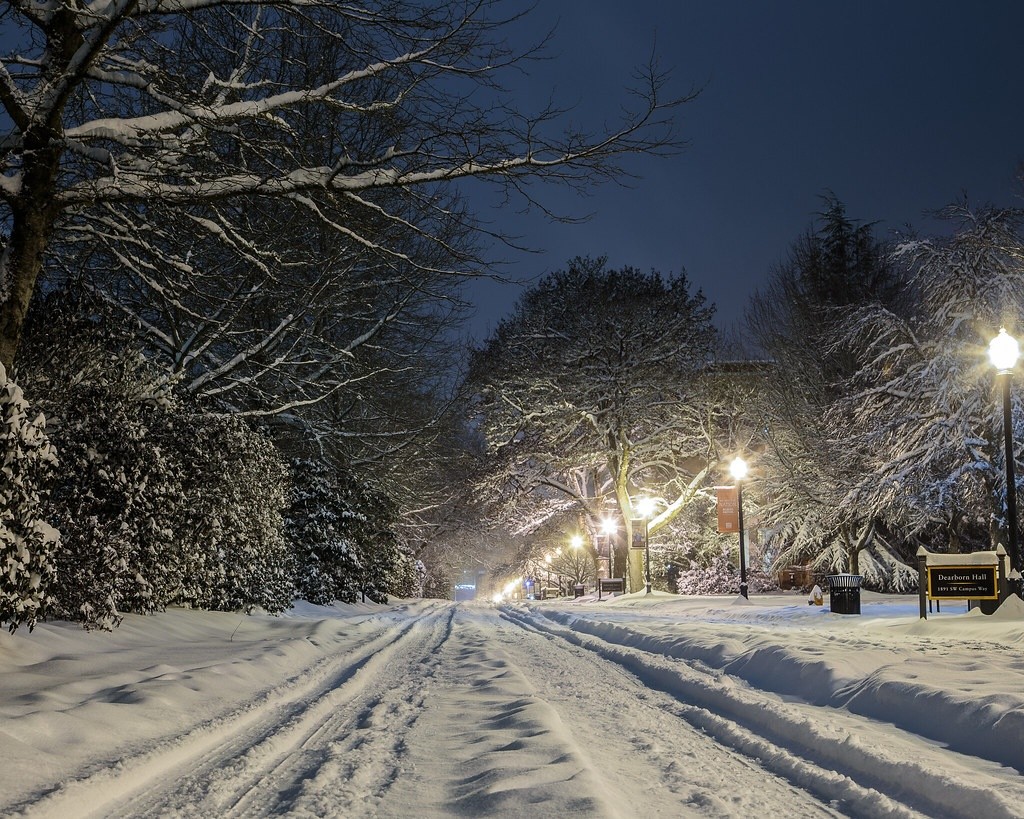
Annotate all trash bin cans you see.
[824,573,864,615]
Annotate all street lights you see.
[988,328,1020,575]
[604,521,619,578]
[730,457,749,598]
[572,535,584,581]
[491,577,524,603]
[545,554,553,587]
[638,497,655,593]
